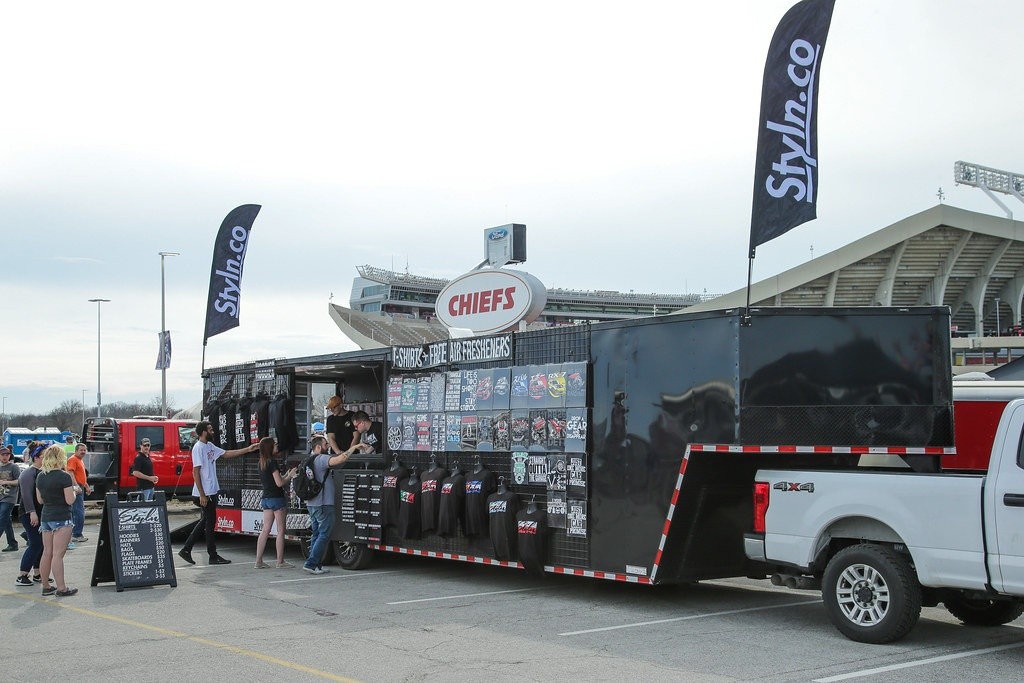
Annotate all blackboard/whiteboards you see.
[90,491,176,586]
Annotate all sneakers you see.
[33,573,54,583]
[209,555,231,564]
[178,549,196,564]
[303,564,329,574]
[15,573,33,586]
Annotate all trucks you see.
[61,417,201,502]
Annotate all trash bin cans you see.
[955,355,966,366]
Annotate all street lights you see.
[88,299,111,418]
[160,251,181,421]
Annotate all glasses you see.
[143,444,149,447]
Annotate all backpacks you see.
[293,454,330,500]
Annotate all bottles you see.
[306,466,314,480]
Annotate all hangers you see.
[389,453,400,471]
[451,460,460,477]
[205,389,290,402]
[408,466,419,485]
[427,454,437,473]
[473,455,482,474]
[526,494,538,514]
[497,476,506,494]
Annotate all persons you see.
[351,410,382,454]
[132,438,158,501]
[178,421,261,565]
[254,437,298,569]
[15,442,54,587]
[35,446,79,597]
[302,437,368,575]
[6,436,76,463]
[0,443,92,552]
[326,396,361,454]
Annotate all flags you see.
[155,330,171,370]
[204,203,263,339]
[750,0,836,249]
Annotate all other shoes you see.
[275,560,295,569]
[42,586,57,596]
[254,561,270,568]
[55,587,79,597]
[2,543,18,551]
[72,535,88,542]
[67,542,76,549]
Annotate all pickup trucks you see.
[743,398,1024,644]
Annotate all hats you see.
[67,436,73,443]
[141,437,150,445]
[0,448,11,454]
[326,396,341,409]
